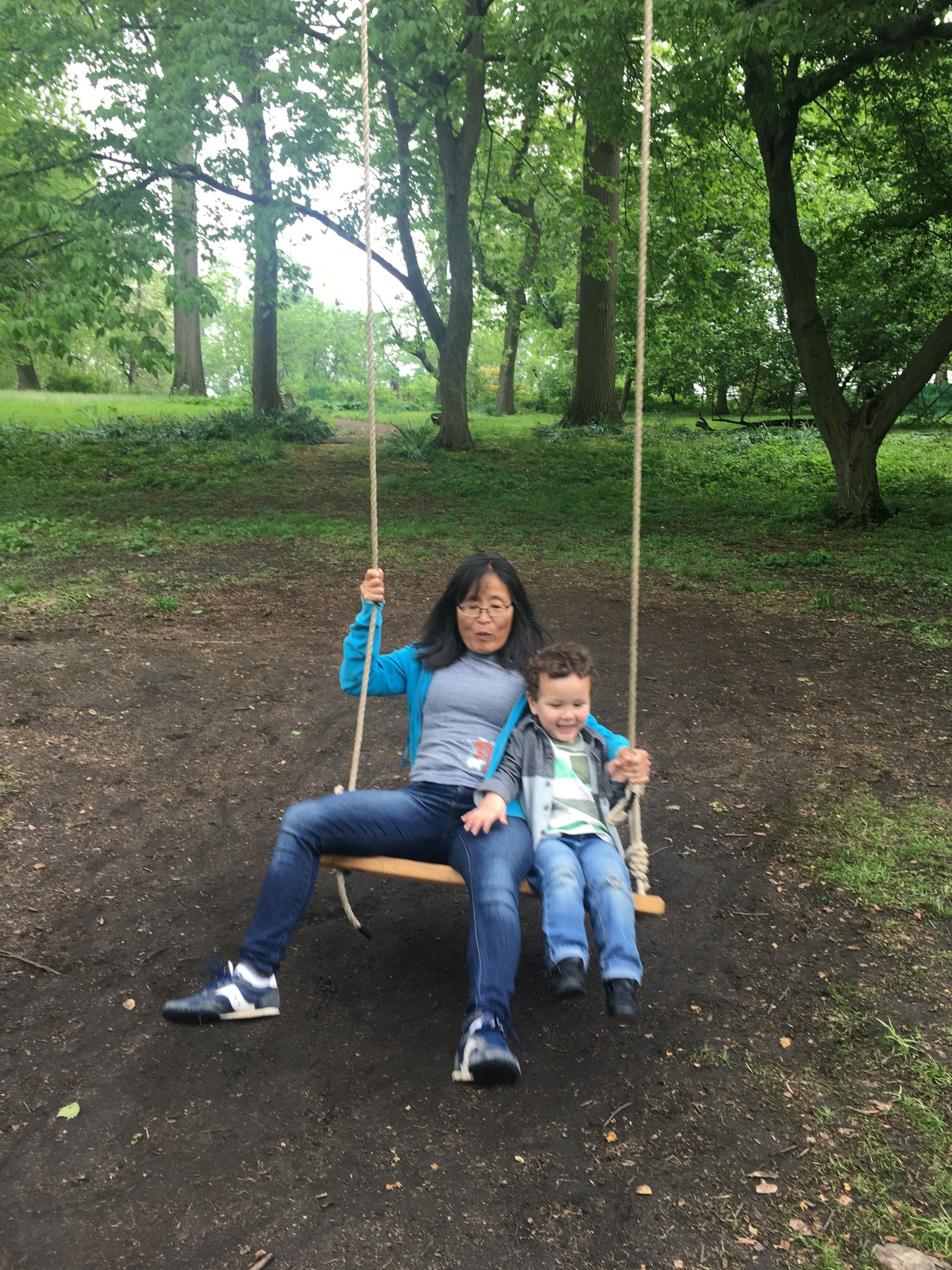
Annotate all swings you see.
[315,0,667,917]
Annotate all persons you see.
[162,550,651,1090]
[461,641,639,1022]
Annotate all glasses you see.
[455,603,513,618]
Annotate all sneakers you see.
[163,960,280,1022]
[550,957,586,1001]
[451,1031,521,1090]
[606,977,640,1017]
